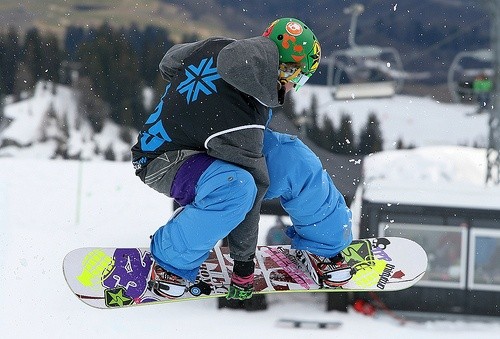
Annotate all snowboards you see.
[63,236,428,312]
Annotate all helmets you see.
[262,18,321,74]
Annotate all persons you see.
[473,72,493,113]
[129,17,352,301]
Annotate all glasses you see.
[295,73,312,92]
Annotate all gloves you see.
[226,260,255,300]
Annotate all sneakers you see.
[295,249,352,286]
[151,259,187,299]
